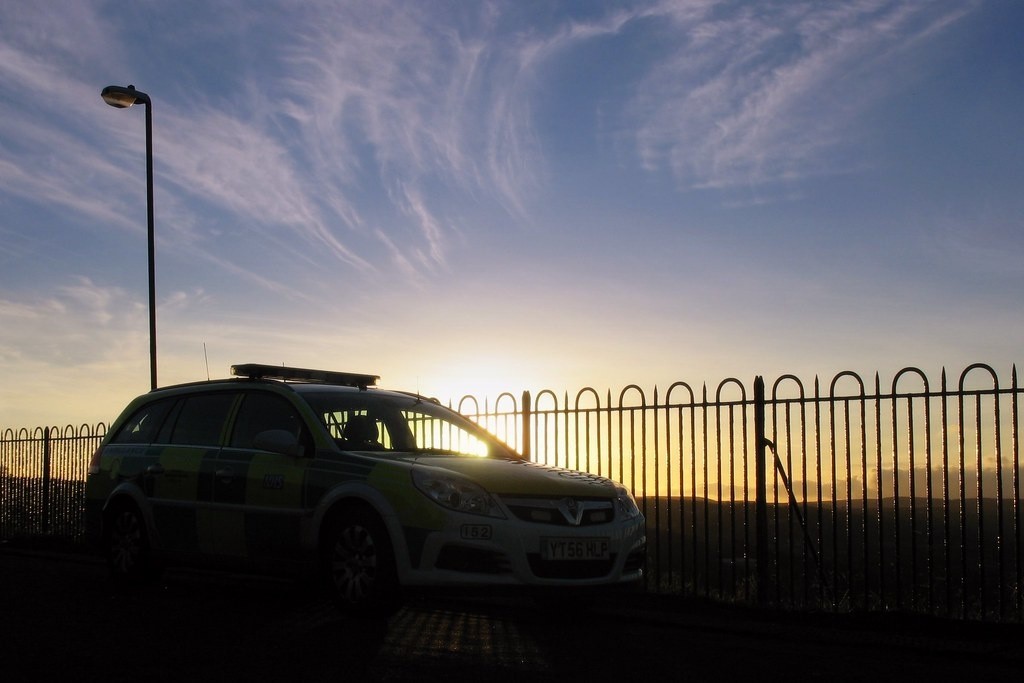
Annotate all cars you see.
[84,363,647,626]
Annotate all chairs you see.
[342,415,383,449]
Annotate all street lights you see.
[98,83,159,393]
